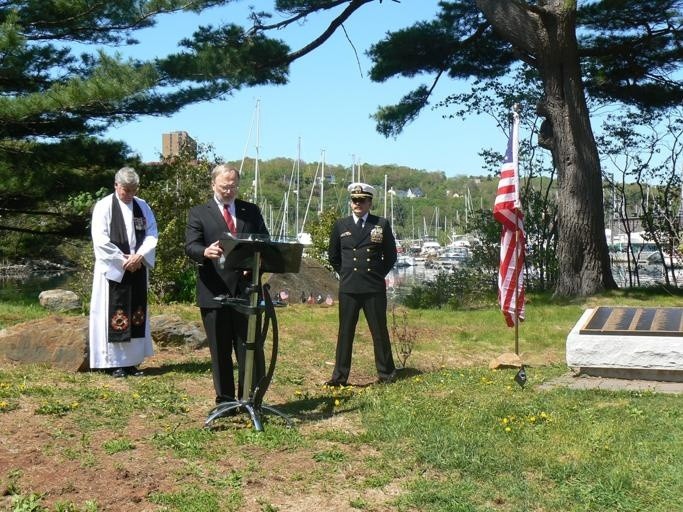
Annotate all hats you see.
[347,183,376,199]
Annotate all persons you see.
[325,183,397,387]
[185,164,271,418]
[90,166,157,377]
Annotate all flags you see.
[493,113,525,327]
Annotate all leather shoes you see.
[122,366,144,376]
[110,367,127,377]
[324,380,346,386]
[207,407,236,419]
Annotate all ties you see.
[223,203,237,237]
[356,216,364,231]
